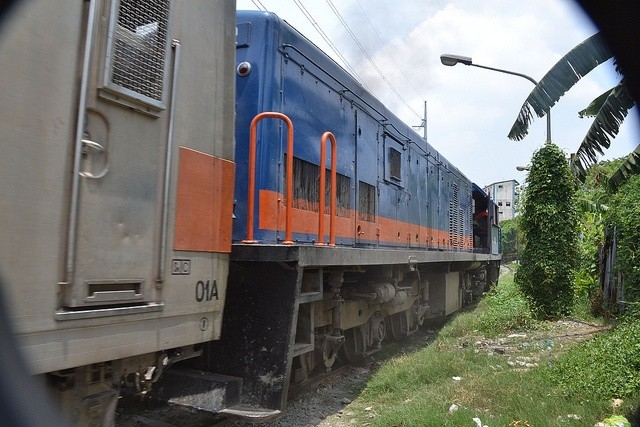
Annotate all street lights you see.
[516,165,530,172]
[440,53,552,148]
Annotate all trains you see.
[0,1,503,427]
